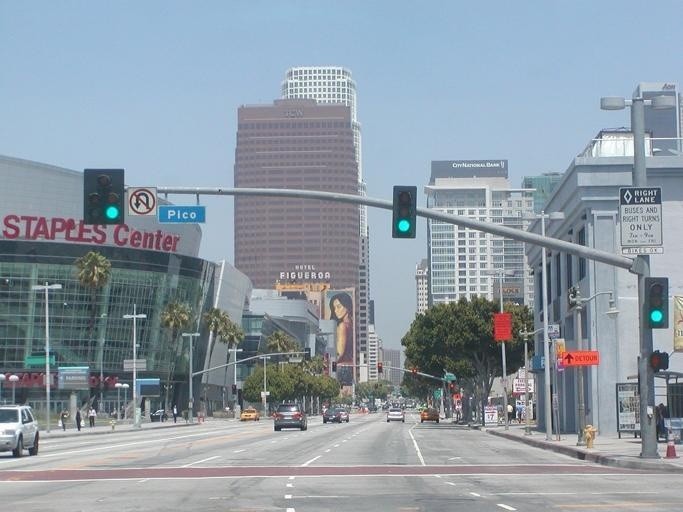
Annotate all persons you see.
[87,406,96,428]
[197,396,204,422]
[173,404,177,423]
[484,398,524,425]
[61,407,69,431]
[454,400,462,421]
[329,292,354,364]
[655,403,666,440]
[75,408,81,431]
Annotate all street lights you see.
[485,266,516,431]
[8,375,19,404]
[598,91,678,461]
[180,331,201,422]
[121,383,129,408]
[227,348,244,405]
[521,209,565,441]
[113,382,122,420]
[567,284,621,449]
[0,373,6,401]
[393,186,416,238]
[31,281,63,434]
[258,355,271,417]
[518,324,552,436]
[122,305,147,426]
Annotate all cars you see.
[334,407,350,422]
[239,405,260,422]
[321,406,340,424]
[141,408,169,422]
[320,394,422,412]
[384,407,406,423]
[419,404,439,423]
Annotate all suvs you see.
[0,404,39,458]
[272,402,309,431]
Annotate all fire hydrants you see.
[583,425,597,449]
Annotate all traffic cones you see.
[663,428,680,459]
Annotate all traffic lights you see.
[645,278,668,328]
[331,362,337,372]
[83,167,123,225]
[377,362,382,373]
[231,384,237,395]
[412,368,417,378]
[651,352,669,370]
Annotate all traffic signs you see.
[433,390,440,399]
[619,187,662,245]
[622,247,665,254]
[444,374,456,380]
[562,351,599,365]
[159,206,205,222]
